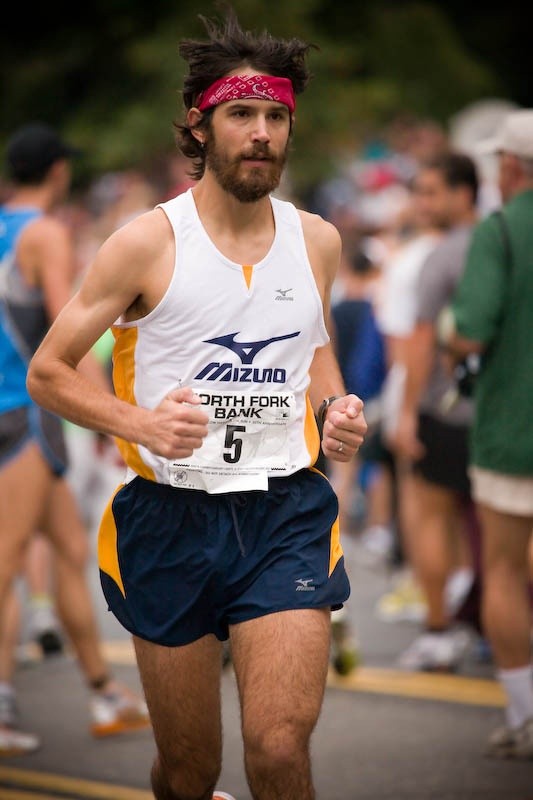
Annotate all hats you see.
[484,108,533,160]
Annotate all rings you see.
[338,441,343,452]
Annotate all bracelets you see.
[318,395,339,431]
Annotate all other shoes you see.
[41,628,65,656]
[400,630,459,672]
[0,690,41,759]
[491,716,533,760]
[332,618,356,677]
[91,690,150,738]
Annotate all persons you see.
[26,22,368,800]
[0,125,202,760]
[303,95,533,760]
